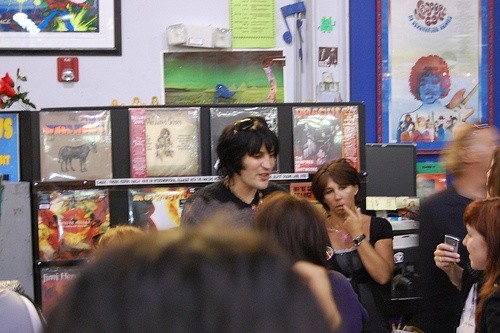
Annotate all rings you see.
[439,257,442,261]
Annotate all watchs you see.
[352,234,366,244]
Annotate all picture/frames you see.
[0,0,121,57]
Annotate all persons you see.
[0,116,500,333]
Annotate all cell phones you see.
[445,235,459,255]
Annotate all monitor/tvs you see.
[365,143,417,217]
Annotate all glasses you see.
[232,115,269,132]
